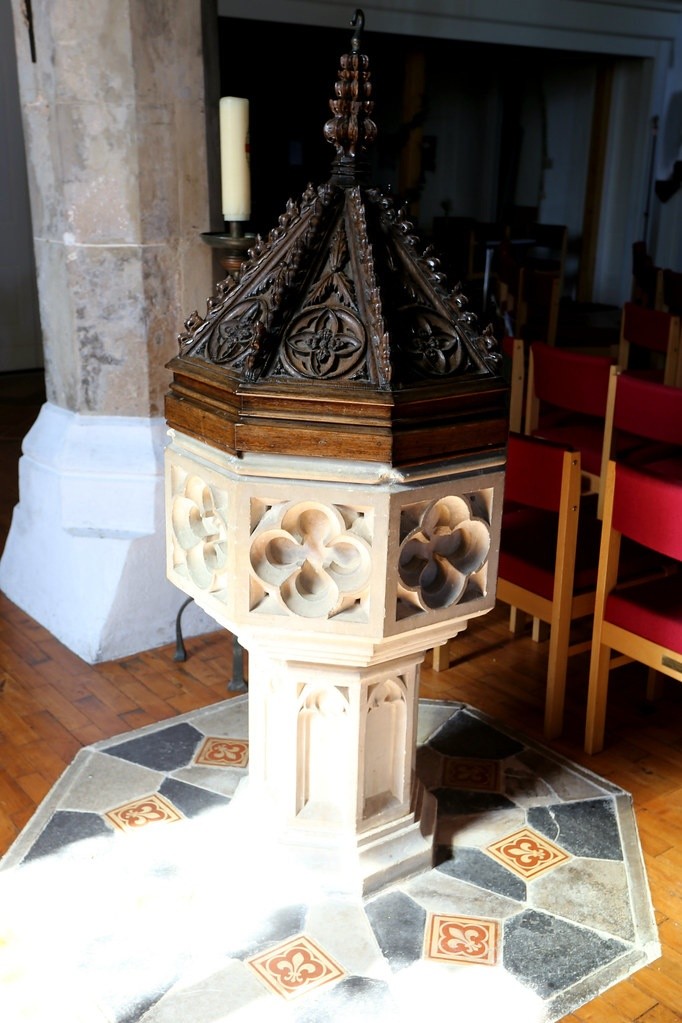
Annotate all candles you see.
[219,96,251,222]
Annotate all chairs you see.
[431,204,682,754]
